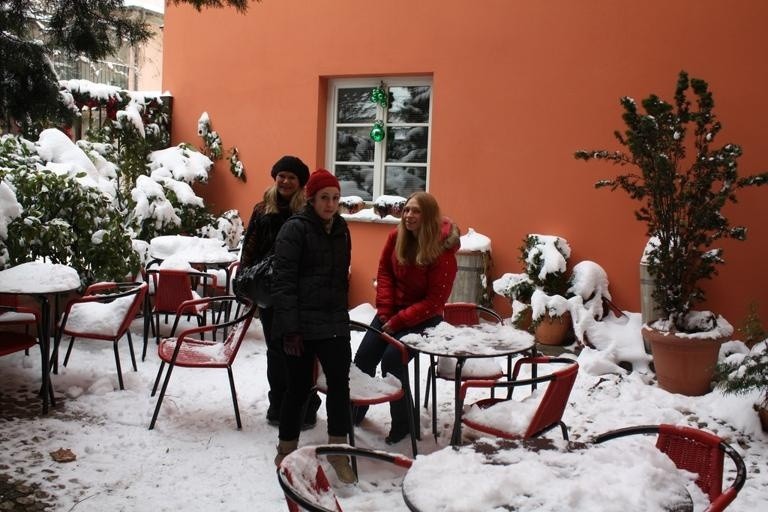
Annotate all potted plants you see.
[573,71,768,396]
[518,233,571,346]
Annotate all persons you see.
[229,155,322,431]
[349,191,461,445]
[272,169,357,484]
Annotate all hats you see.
[305,168,341,198]
[271,155,310,188]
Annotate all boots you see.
[384,396,417,446]
[327,434,358,485]
[353,405,369,425]
[275,438,298,467]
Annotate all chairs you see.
[145,260,168,333]
[310,319,418,457]
[425,303,511,437]
[0,309,57,407]
[278,442,413,509]
[148,296,258,432]
[50,283,148,391]
[587,424,747,511]
[142,270,220,344]
[451,356,579,447]
[214,261,248,334]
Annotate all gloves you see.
[231,278,248,306]
[282,333,304,355]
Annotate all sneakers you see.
[300,387,322,432]
[266,389,280,427]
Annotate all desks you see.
[402,436,694,512]
[399,325,536,448]
[0,277,80,414]
[151,254,237,329]
